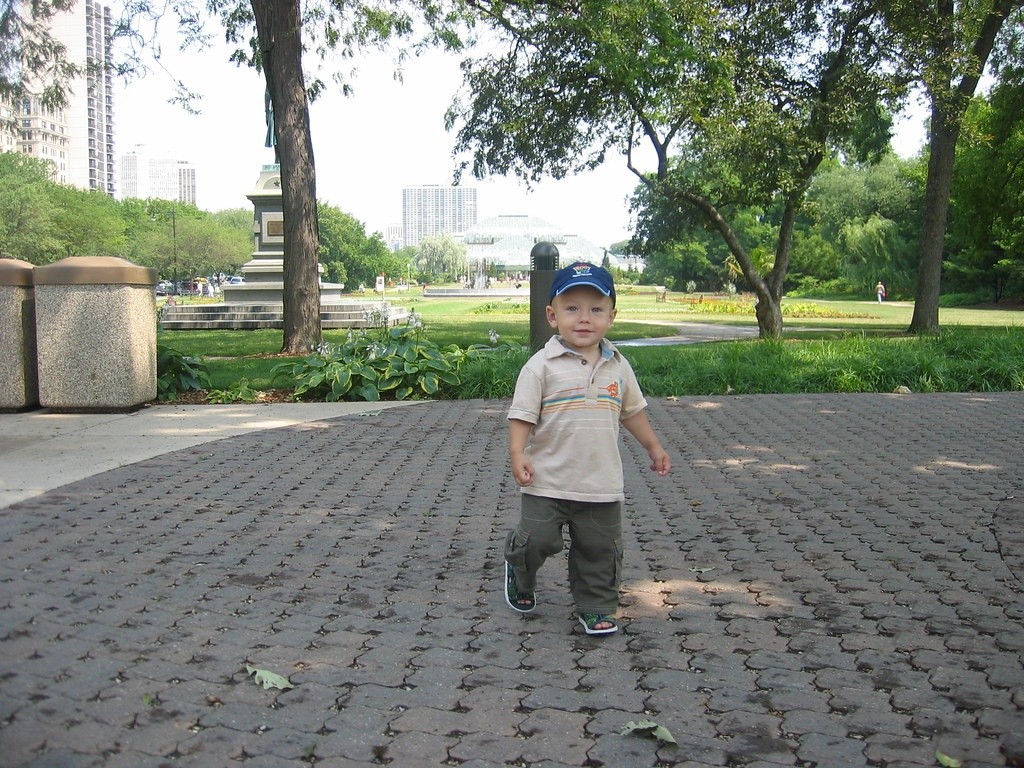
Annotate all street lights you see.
[407,264,410,291]
[467,261,470,289]
[151,207,178,296]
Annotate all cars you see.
[203,283,214,297]
[179,280,201,297]
[212,273,225,282]
[156,280,174,296]
[230,277,245,284]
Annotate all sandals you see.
[578,612,618,634]
[504,559,536,612]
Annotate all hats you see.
[550,262,615,305]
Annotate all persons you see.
[208,283,214,297]
[423,282,428,293]
[196,280,203,296]
[359,283,365,293]
[504,261,670,633]
[514,278,520,289]
[875,281,886,304]
